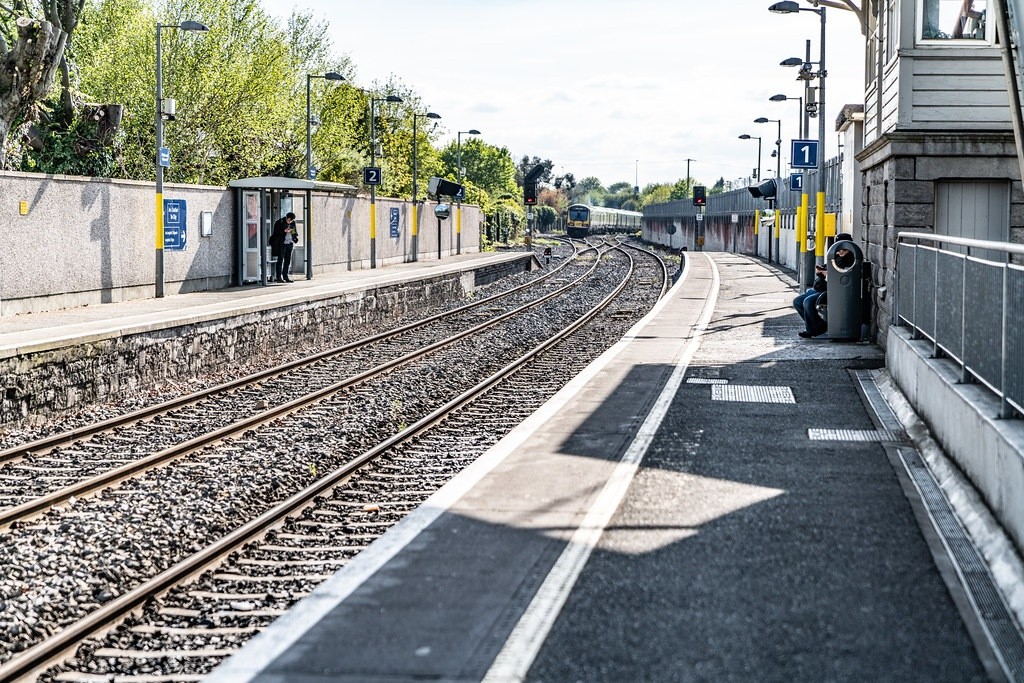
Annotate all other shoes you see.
[277,279,284,283]
[286,279,293,282]
[799,331,812,337]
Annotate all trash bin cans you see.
[826,239,864,338]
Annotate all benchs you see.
[811,291,871,342]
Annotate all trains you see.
[566,204,644,238]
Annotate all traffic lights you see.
[525,196,538,205]
[545,248,551,256]
[693,186,706,206]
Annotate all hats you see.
[836,233,853,241]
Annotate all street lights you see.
[156,21,211,298]
[739,135,762,183]
[767,0,826,282]
[371,96,403,268]
[769,94,802,285]
[413,112,441,261]
[636,159,639,187]
[307,73,346,282]
[684,159,697,199]
[779,39,812,288]
[457,130,481,254]
[753,118,782,266]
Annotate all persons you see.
[268,212,298,283]
[793,233,853,339]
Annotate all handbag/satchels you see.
[269,235,276,245]
[813,276,827,292]
